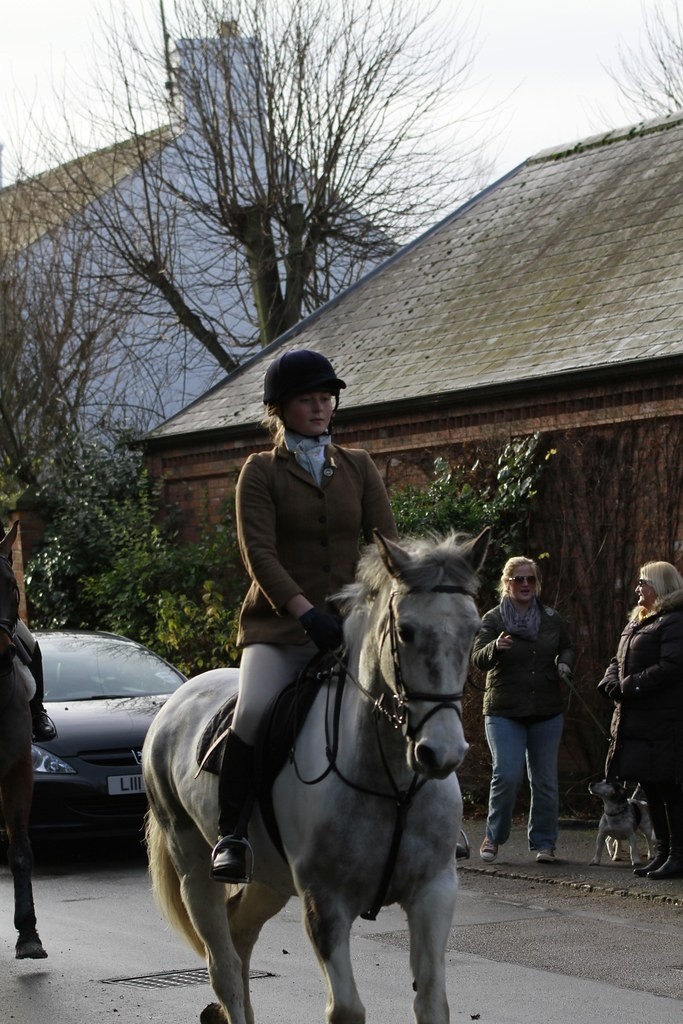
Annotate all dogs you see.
[588,776,657,868]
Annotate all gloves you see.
[605,680,624,702]
[297,607,344,654]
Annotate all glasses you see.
[638,579,652,587]
[508,575,536,585]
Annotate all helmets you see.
[263,349,346,406]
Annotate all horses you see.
[140,525,493,1024]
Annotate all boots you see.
[210,728,266,884]
[632,839,670,875]
[647,844,683,878]
[28,640,58,742]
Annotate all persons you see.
[210,351,467,884]
[596,562,683,878]
[470,557,574,862]
[0,519,55,739]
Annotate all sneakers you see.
[479,837,499,862]
[536,848,555,862]
